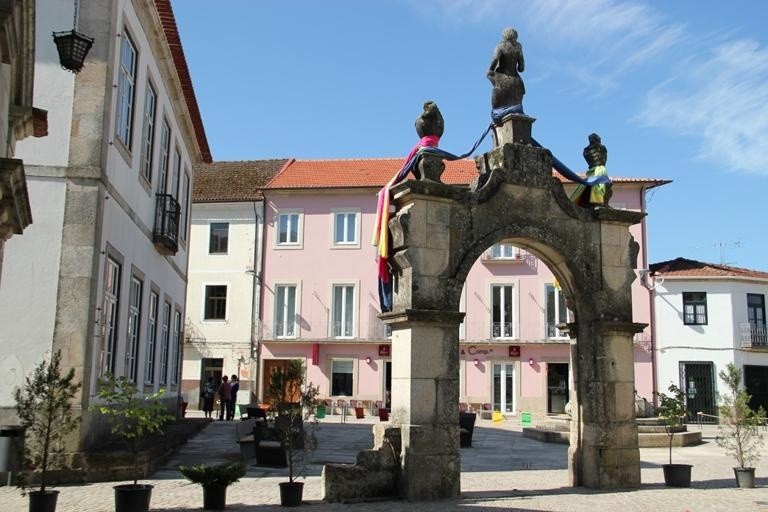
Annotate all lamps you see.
[49,0,96,75]
[366,356,371,364]
[474,359,479,366]
[529,358,534,365]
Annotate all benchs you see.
[459,411,477,449]
[235,398,306,468]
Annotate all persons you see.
[200,374,239,422]
[582,133,614,205]
[414,100,444,139]
[485,27,526,108]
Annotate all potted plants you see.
[178,454,250,511]
[257,357,329,508]
[652,381,695,488]
[713,361,768,488]
[13,348,86,512]
[86,368,177,512]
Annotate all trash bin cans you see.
[317,405,326,418]
[0,424,26,486]
[459,413,476,448]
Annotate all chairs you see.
[459,403,492,419]
[303,398,382,417]
